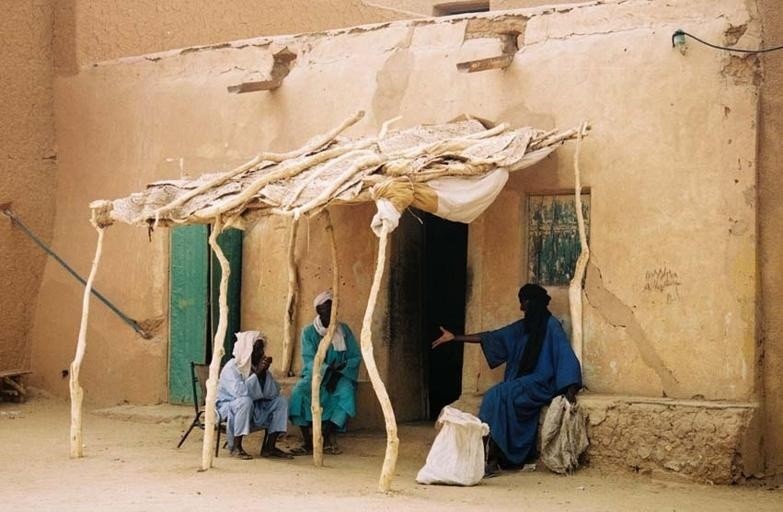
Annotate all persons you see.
[432,282,582,468]
[290,290,360,454]
[216,330,289,460]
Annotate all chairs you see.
[177,361,268,458]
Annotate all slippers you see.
[231,444,342,459]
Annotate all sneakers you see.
[481,461,500,478]
